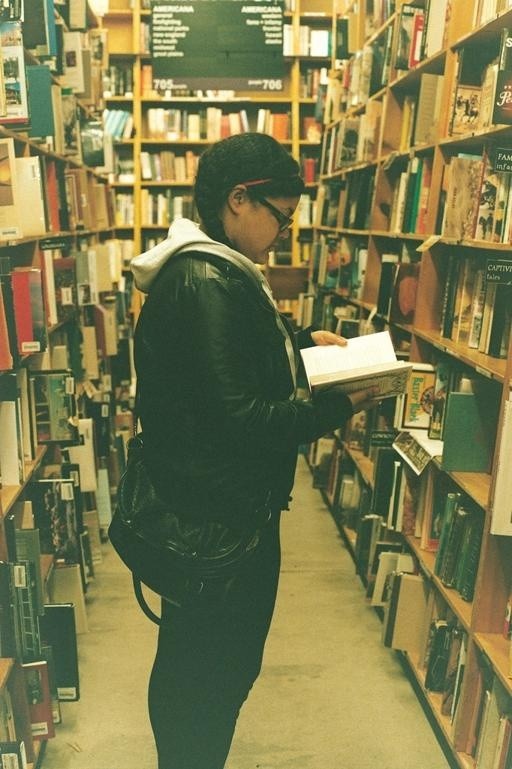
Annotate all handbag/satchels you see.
[106,431,275,612]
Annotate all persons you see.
[110,127,382,769]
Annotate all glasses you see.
[247,186,294,233]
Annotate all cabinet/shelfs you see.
[1,1,141,768]
[86,1,348,331]
[300,3,512,767]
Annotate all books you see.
[300,330,413,402]
[139,108,323,312]
[305,0,511,769]
[0,0,144,769]
[293,320,512,769]
